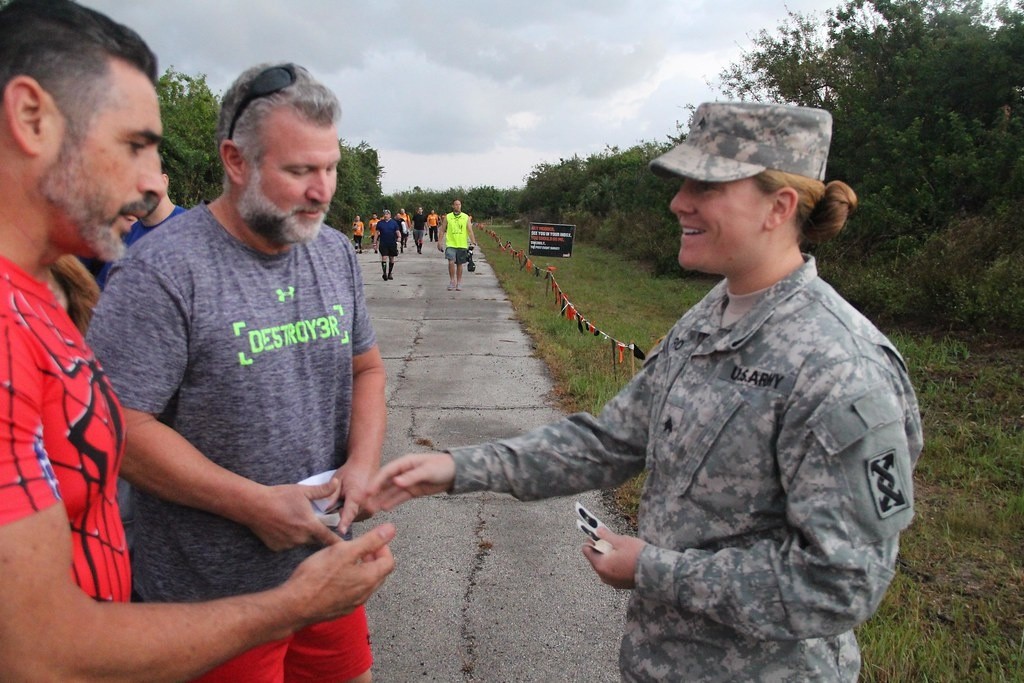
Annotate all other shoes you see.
[420,250,421,254]
[383,274,388,280]
[417,249,419,252]
[388,273,393,279]
[447,283,456,289]
[456,284,462,290]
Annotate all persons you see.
[50,160,188,340]
[369,208,410,281]
[87,64,386,683]
[352,216,364,254]
[0,0,397,683]
[412,206,447,254]
[438,200,475,290]
[366,101,924,683]
[470,216,473,223]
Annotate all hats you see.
[649,102,833,183]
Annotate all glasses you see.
[228,64,295,140]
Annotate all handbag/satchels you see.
[468,250,476,271]
